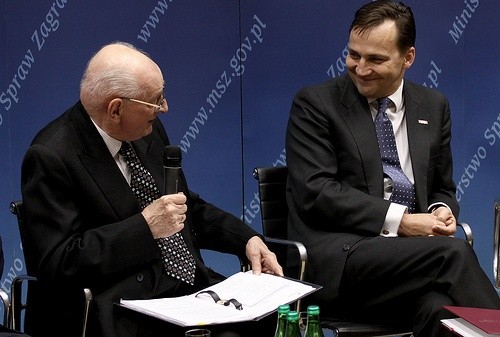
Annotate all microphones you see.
[162,144,182,196]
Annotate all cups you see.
[185,329,211,337]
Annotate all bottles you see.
[303,306,325,337]
[274,305,290,337]
[283,311,302,337]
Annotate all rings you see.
[181,216,183,221]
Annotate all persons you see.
[285,0,500,337]
[20,42,283,337]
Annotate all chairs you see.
[0,288,10,328]
[252,166,473,337]
[10,200,253,337]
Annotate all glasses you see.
[117,88,165,114]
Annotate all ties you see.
[118,140,196,286]
[373,97,415,214]
